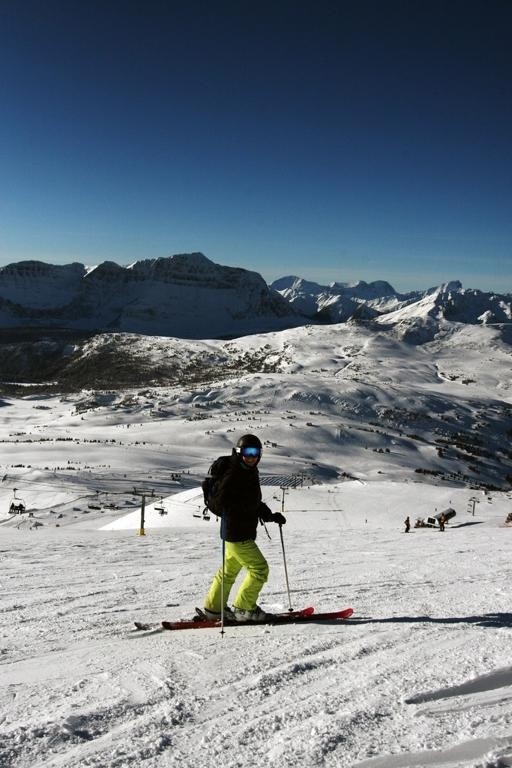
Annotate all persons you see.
[439,513,446,532]
[404,516,411,533]
[201,434,286,621]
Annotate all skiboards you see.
[134,607,353,630]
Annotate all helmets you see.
[236,435,262,464]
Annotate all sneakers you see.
[233,605,266,621]
[206,607,234,620]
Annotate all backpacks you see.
[202,455,233,517]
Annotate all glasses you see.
[236,447,263,458]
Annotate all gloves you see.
[270,512,286,524]
